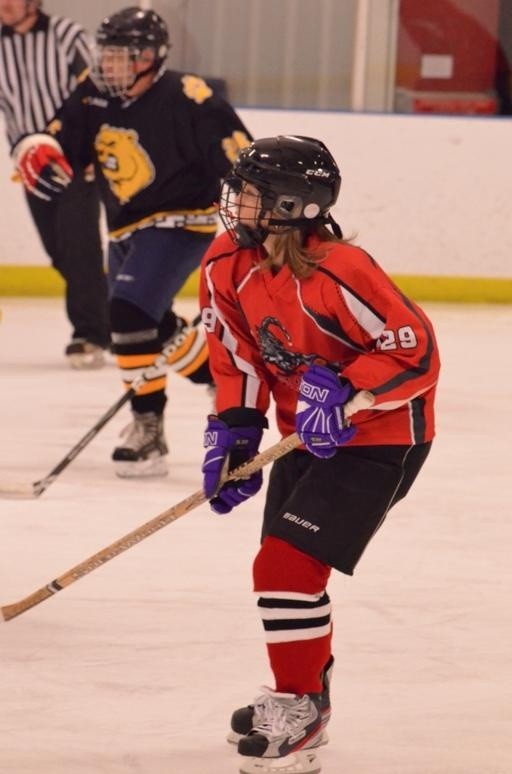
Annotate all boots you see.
[231,654,333,757]
[112,410,169,460]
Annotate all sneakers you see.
[66,338,99,355]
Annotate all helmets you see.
[219,136,341,249]
[89,7,168,97]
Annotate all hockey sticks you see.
[1,316,203,497]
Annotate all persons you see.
[10,2,256,472]
[196,131,443,760]
[0,0,110,360]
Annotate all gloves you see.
[203,408,268,514]
[10,133,74,200]
[297,366,356,459]
